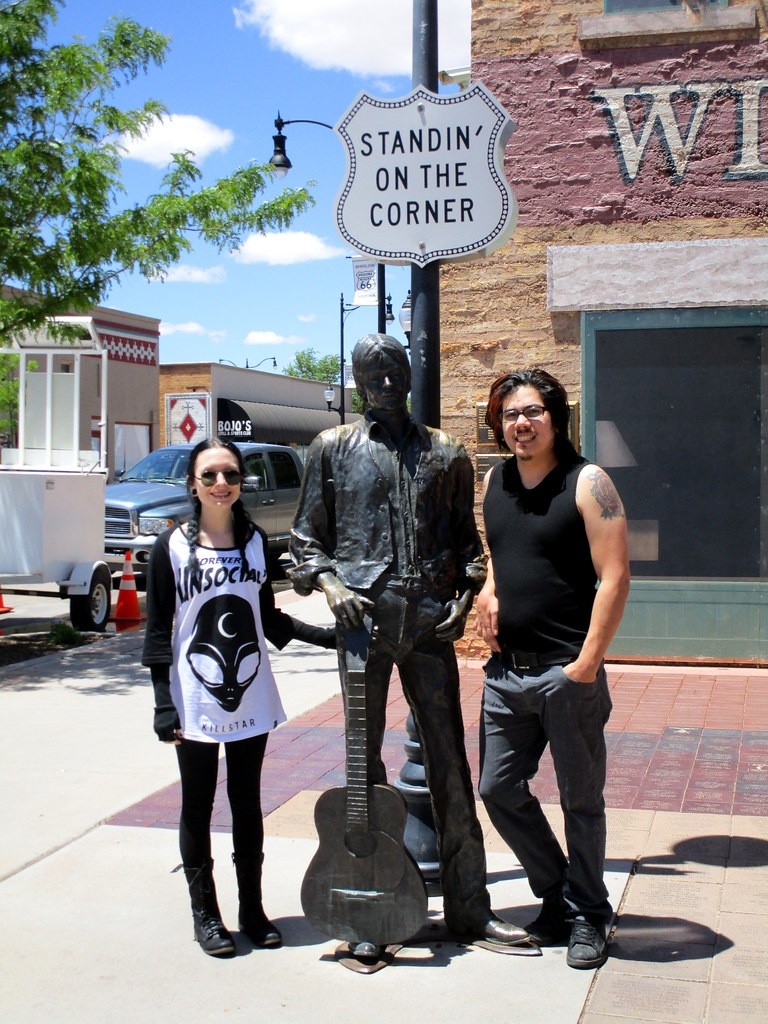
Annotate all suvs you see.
[105,441,305,576]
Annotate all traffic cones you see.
[0,593,15,614]
[107,550,146,621]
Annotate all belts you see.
[500,651,575,669]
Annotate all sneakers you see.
[567,918,608,967]
[523,901,574,941]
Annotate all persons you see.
[286,333,532,959]
[473,368,630,968]
[141,436,337,956]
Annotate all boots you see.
[231,852,282,945]
[182,858,235,953]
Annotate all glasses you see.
[194,471,243,487]
[499,404,548,423]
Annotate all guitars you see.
[300,606,429,943]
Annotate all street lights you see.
[269,108,412,356]
[325,290,393,425]
